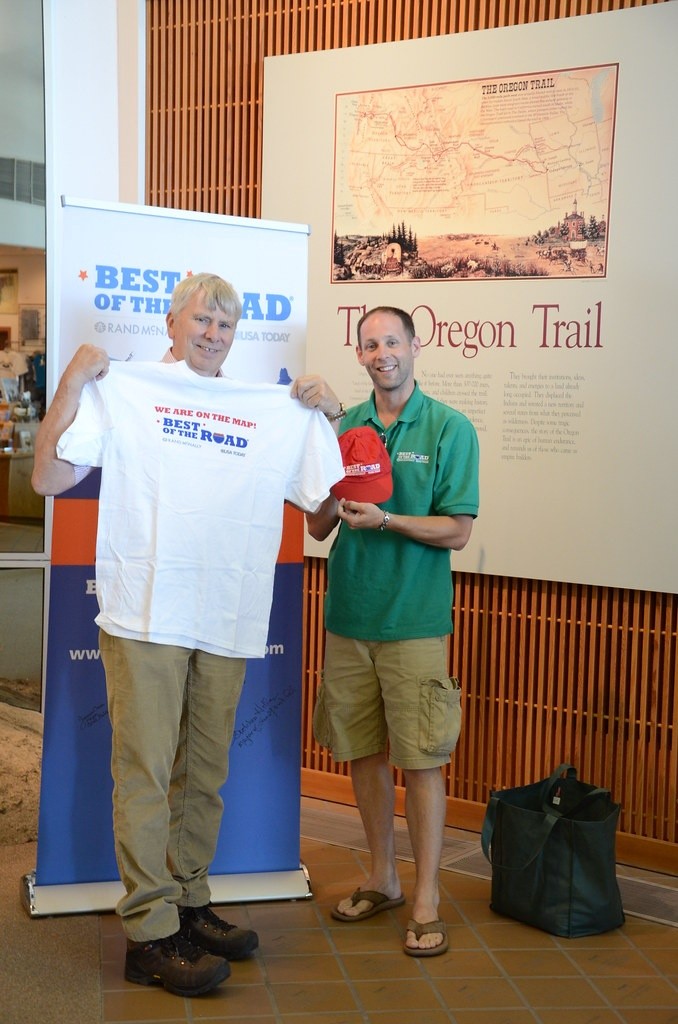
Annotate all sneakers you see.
[171,904,259,961]
[124,931,232,997]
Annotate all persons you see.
[309,307,482,960]
[31,272,345,999]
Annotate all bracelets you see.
[377,511,390,534]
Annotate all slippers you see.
[330,885,406,923]
[401,915,449,957]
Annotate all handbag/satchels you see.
[480,762,626,939]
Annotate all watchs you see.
[326,403,346,421]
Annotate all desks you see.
[0,450,44,520]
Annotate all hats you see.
[328,425,393,510]
